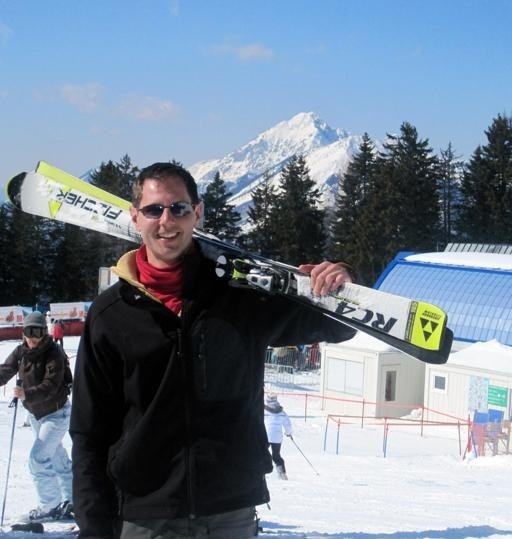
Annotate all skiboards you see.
[6,161,453,362]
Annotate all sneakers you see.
[28,503,62,521]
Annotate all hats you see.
[22,311,47,327]
[266,393,277,403]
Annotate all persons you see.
[43,311,66,349]
[66,161,355,538]
[1,309,74,531]
[258,391,293,476]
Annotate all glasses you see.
[23,326,46,338]
[138,201,193,219]
